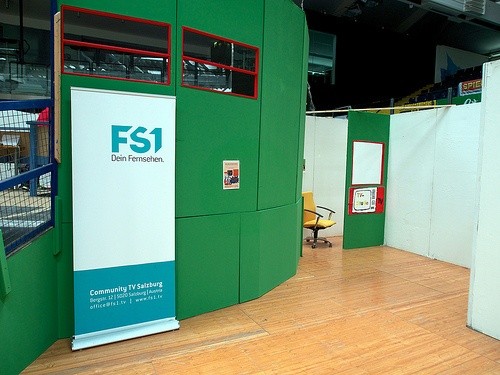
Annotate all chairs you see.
[301,191,336,248]
